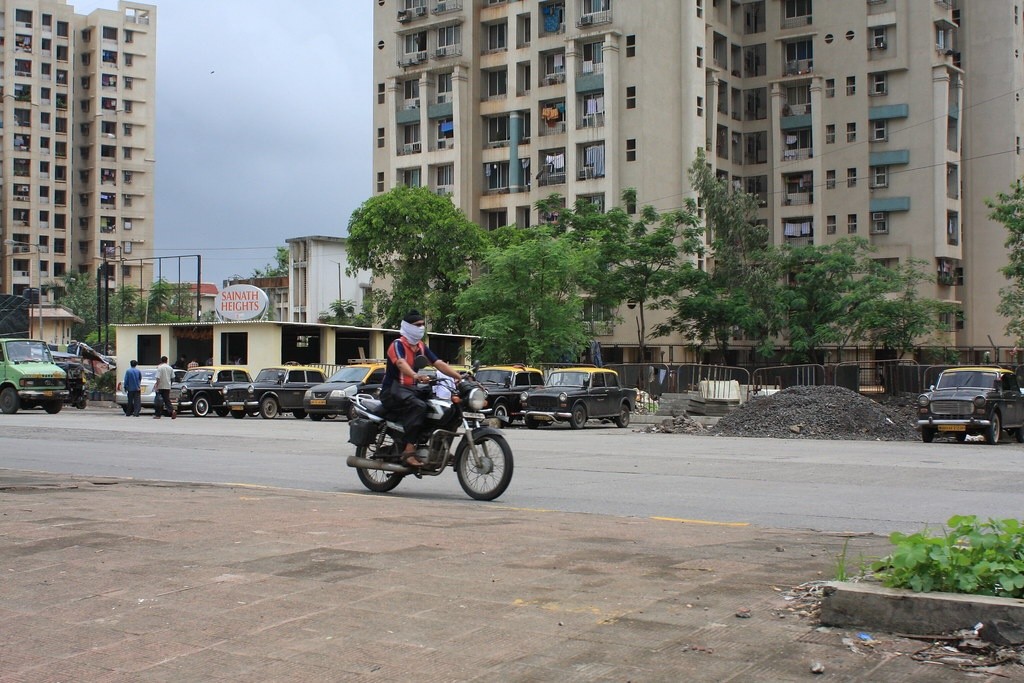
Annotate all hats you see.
[403,309,423,324]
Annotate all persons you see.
[152,357,176,420]
[124,359,142,417]
[171,353,243,371]
[647,361,655,395]
[379,309,461,468]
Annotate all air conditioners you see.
[873,212,883,220]
[415,6,425,15]
[416,51,426,60]
[437,48,446,56]
[412,143,421,152]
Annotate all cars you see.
[520,367,637,429]
[415,364,472,382]
[115,368,187,414]
[466,363,543,426]
[222,363,329,418]
[918,366,1024,445]
[170,365,255,419]
[304,357,387,421]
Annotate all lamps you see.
[580,15,593,25]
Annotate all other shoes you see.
[153,416,160,419]
[171,410,177,419]
[399,451,424,468]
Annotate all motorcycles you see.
[56,362,86,409]
[346,360,514,500]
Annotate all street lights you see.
[4,239,43,339]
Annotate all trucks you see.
[0,335,67,415]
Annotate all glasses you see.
[412,322,425,327]
